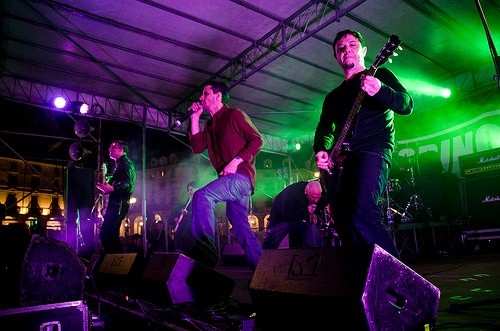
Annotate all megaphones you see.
[69,142,92,161]
[74,119,94,138]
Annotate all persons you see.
[313,29,415,310]
[187,80,262,272]
[175,182,200,242]
[262,181,328,249]
[96,140,136,255]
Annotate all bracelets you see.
[235,157,240,164]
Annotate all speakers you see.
[66,167,94,254]
[462,176,500,229]
[91,251,236,307]
[249,244,441,331]
[18,233,87,305]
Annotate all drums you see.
[410,194,423,207]
[385,179,401,192]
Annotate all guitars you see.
[320,34,403,203]
[97,163,107,219]
[168,197,191,240]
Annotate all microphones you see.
[175,109,194,126]
